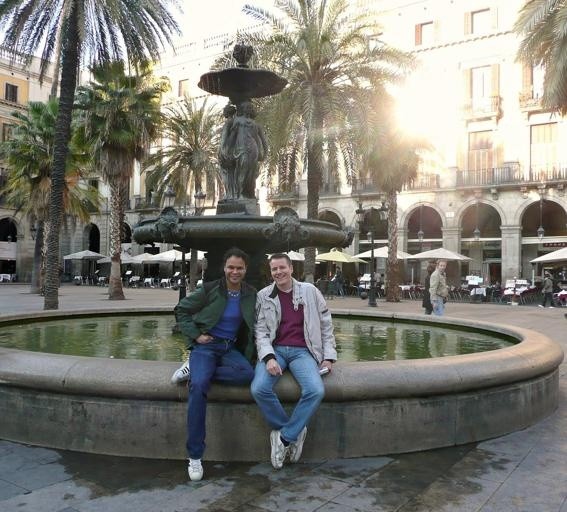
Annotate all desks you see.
[196,279,203,288]
[74,275,82,285]
[0,274,16,283]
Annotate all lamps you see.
[355,202,366,225]
[377,200,389,221]
[417,203,424,244]
[29,223,37,241]
[7,217,12,243]
[366,227,373,245]
[474,189,480,242]
[537,185,546,241]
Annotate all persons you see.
[246,106,267,196]
[63,269,190,290]
[172,246,258,481]
[420,262,436,315]
[428,260,449,315]
[223,102,262,201]
[312,265,566,311]
[216,104,235,201]
[247,253,339,471]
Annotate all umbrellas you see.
[349,245,410,258]
[312,250,368,263]
[528,245,567,263]
[63,248,208,262]
[265,249,320,264]
[404,247,470,259]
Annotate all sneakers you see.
[269,429,290,469]
[170,359,191,385]
[538,305,555,309]
[289,425,308,464]
[186,457,204,481]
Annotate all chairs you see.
[318,271,567,308]
[81,274,190,290]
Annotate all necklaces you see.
[227,288,240,298]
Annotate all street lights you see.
[164,186,206,302]
[355,199,388,306]
